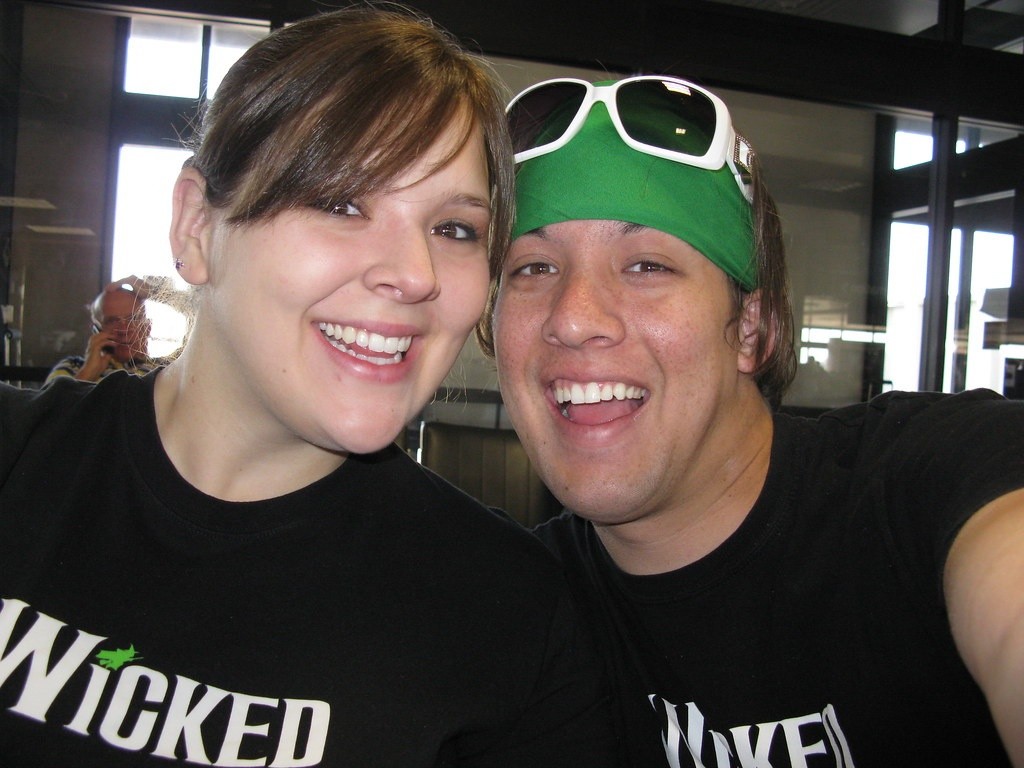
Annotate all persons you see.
[480,77,1024,768]
[41,275,192,386]
[0,0,620,768]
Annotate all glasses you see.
[504,75,756,205]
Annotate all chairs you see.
[420,421,554,530]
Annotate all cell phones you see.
[92,325,107,351]
